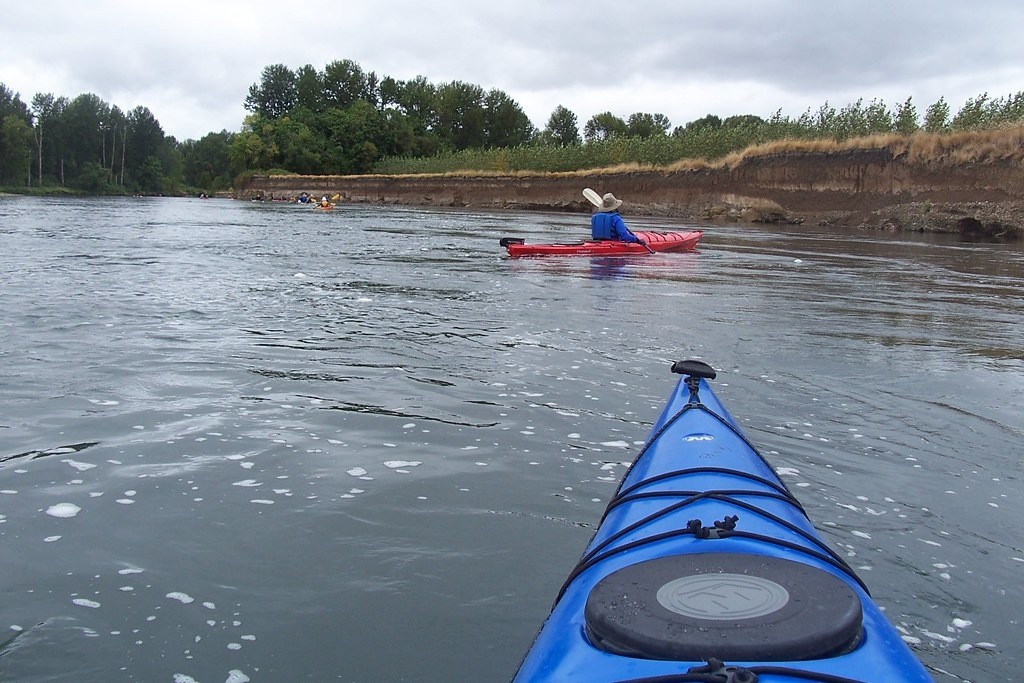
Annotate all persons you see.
[591,193,645,244]
[200,192,331,207]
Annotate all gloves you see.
[636,239,646,245]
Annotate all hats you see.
[599,193,622,212]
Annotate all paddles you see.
[582,188,655,254]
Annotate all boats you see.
[497,230,704,257]
